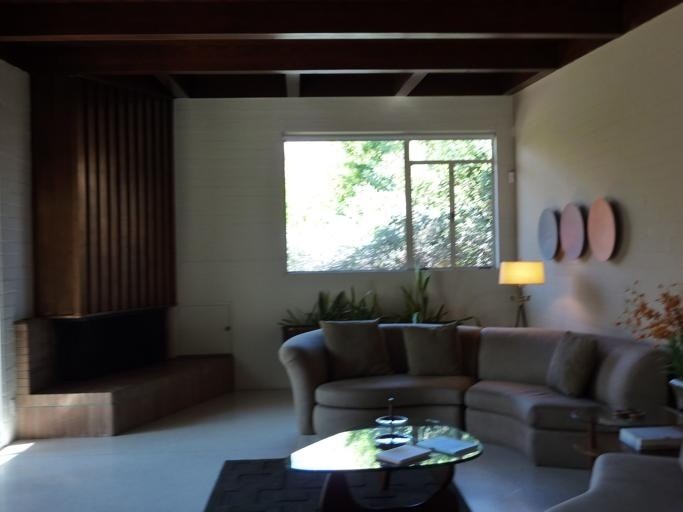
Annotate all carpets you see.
[202,458,472,512]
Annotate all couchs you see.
[278,326,670,468]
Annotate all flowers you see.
[612,279,681,380]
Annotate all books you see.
[375,445,432,464]
[417,436,478,456]
[618,425,683,451]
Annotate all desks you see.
[618,426,681,459]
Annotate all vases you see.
[667,378,681,412]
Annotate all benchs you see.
[14,316,233,439]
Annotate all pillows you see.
[317,317,466,381]
[544,330,597,397]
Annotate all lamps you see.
[499,261,544,328]
